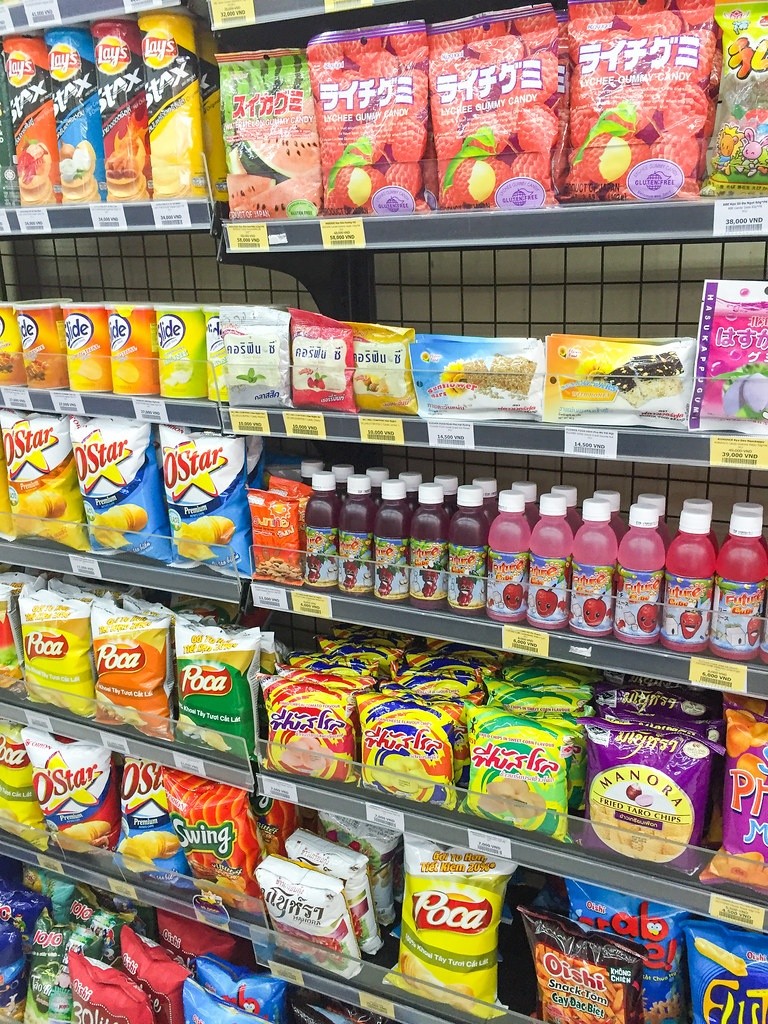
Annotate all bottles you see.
[304,472,340,592]
[486,490,530,623]
[551,486,585,590]
[399,472,421,516]
[374,479,410,603]
[410,485,450,610]
[301,459,323,486]
[338,477,375,598]
[448,486,487,618]
[660,499,768,667]
[366,467,389,508]
[594,490,631,622]
[613,503,665,645]
[638,493,670,628]
[526,493,573,629]
[569,497,618,636]
[333,465,354,502]
[512,482,541,534]
[472,477,498,525]
[434,475,458,521]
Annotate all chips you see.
[176,516,234,560]
[92,503,148,549]
[193,874,247,908]
[400,952,475,1007]
[14,490,66,532]
[59,821,112,848]
[119,830,180,871]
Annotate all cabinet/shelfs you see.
[207,0,767,1023]
[0,0,271,948]
